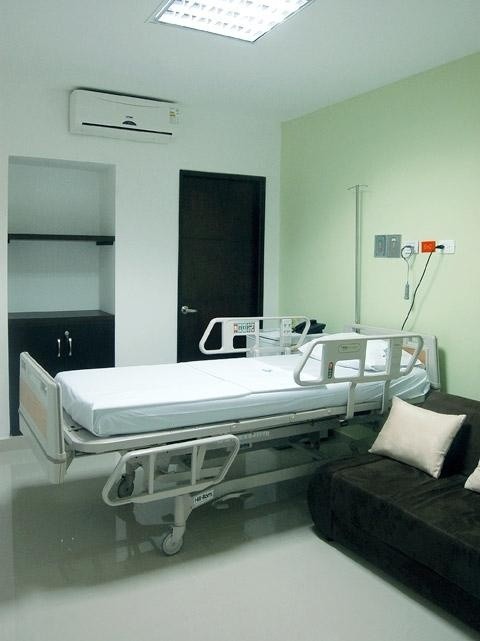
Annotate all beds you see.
[18,326,443,556]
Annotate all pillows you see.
[370,397,468,479]
[463,459,480,494]
[297,330,424,371]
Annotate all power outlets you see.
[438,240,455,255]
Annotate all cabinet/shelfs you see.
[8,310,115,439]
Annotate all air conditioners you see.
[69,87,182,146]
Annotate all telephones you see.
[295,319,326,334]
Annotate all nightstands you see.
[245,328,329,360]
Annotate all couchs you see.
[306,391,480,634]
